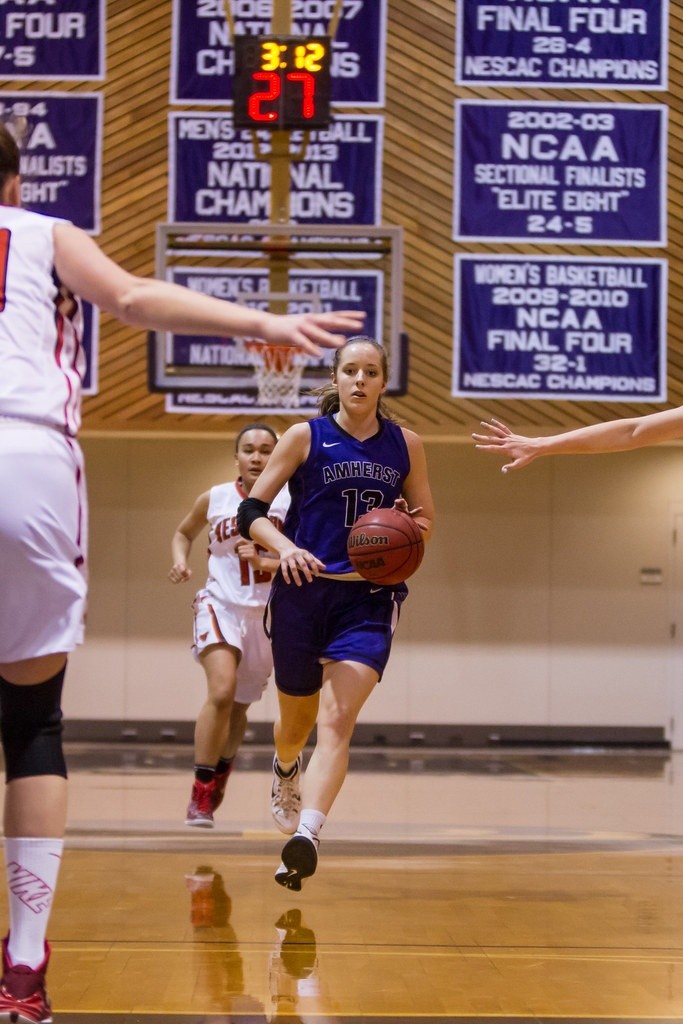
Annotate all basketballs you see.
[347,507,424,586]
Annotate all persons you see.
[471,408,683,477]
[238,336,437,894]
[169,423,293,828]
[0,126,379,1024]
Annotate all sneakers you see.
[275,823,320,892]
[214,762,233,810]
[0,929,52,1024]
[185,779,215,828]
[189,864,232,929]
[274,908,316,978]
[271,750,303,836]
